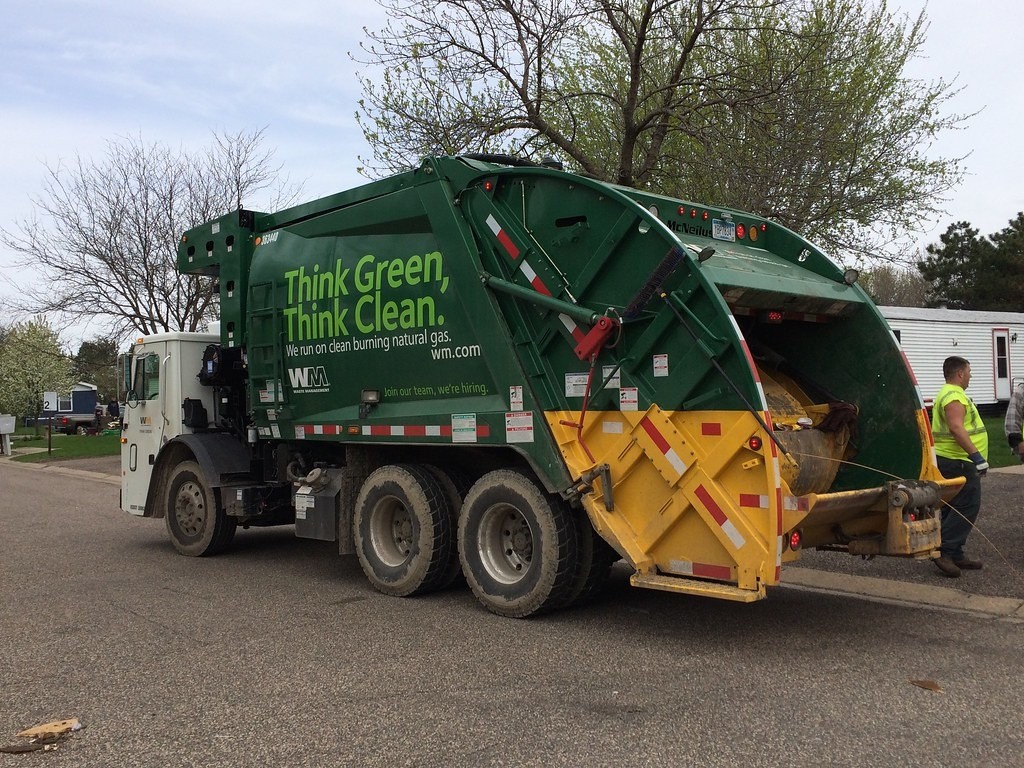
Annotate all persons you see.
[1005,382,1024,464]
[931,356,989,578]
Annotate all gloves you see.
[967,451,990,481]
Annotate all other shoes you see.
[952,556,983,569]
[930,557,961,577]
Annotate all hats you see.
[111,394,117,398]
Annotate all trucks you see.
[117,153,967,618]
[52,405,125,438]
